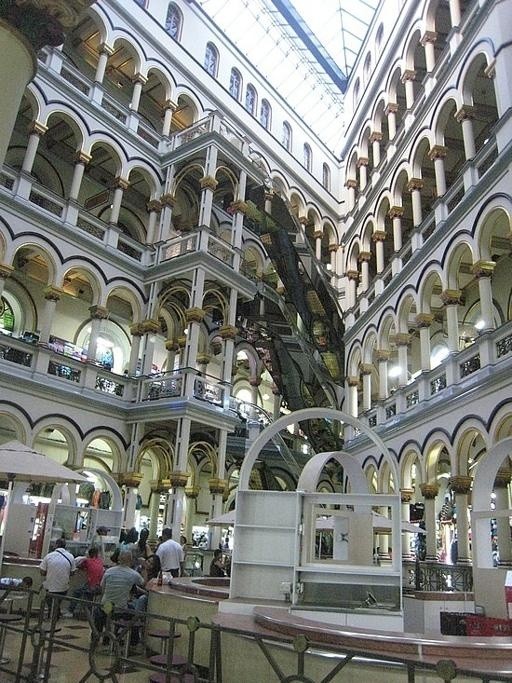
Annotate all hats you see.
[97,526,110,530]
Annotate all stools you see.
[111,620,196,683]
[76,591,102,621]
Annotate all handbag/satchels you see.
[110,547,120,562]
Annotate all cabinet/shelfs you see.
[403,595,476,636]
[217,486,405,632]
[40,504,124,559]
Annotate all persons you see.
[75,510,233,588]
[1,576,32,604]
[97,550,149,641]
[38,538,77,615]
[371,335,479,425]
[437,533,499,566]
[132,554,163,639]
[63,547,107,617]
[1,328,273,428]
[370,542,393,565]
